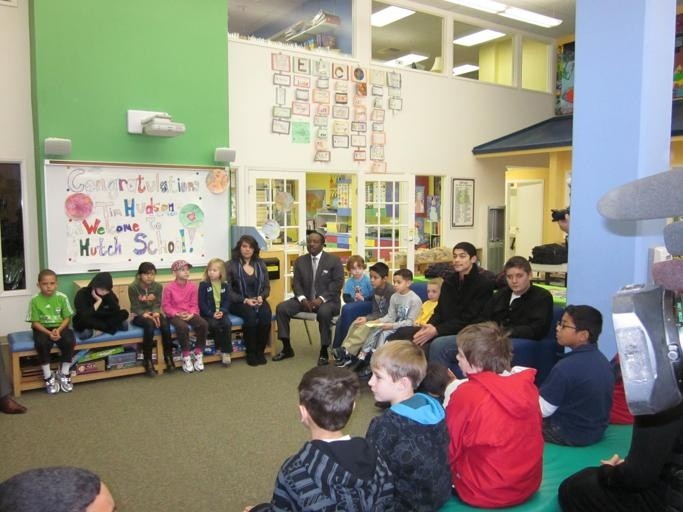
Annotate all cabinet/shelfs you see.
[528,260,567,306]
[316,208,350,249]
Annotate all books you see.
[267,8,341,50]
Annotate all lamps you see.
[371,5,416,28]
[453,27,506,48]
[449,0,564,29]
[384,50,430,67]
[453,63,480,77]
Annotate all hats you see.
[306,226,327,238]
[171,259,192,272]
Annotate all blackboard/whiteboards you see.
[43,159,231,277]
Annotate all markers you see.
[88,269,100,271]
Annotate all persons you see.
[538,305,615,447]
[243,365,395,512]
[164,231,553,409]
[25,269,76,395]
[128,262,176,378]
[0,342,27,415]
[72,272,129,340]
[365,339,454,511]
[0,466,117,512]
[555,211,570,236]
[558,379,683,512]
[445,320,545,509]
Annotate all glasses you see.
[557,321,577,330]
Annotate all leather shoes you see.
[164,354,175,373]
[246,352,258,366]
[143,359,156,377]
[256,352,267,364]
[0,396,28,414]
[272,348,294,361]
[317,351,329,366]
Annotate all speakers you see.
[214,147,236,162]
[44,138,71,156]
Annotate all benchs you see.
[292,310,342,344]
[431,409,635,511]
[6,310,277,397]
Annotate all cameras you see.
[550,207,570,222]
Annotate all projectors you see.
[144,122,185,137]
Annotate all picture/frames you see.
[270,52,403,174]
[452,178,475,227]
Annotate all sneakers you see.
[191,349,204,372]
[56,369,75,393]
[180,351,194,373]
[331,345,372,379]
[222,352,232,365]
[42,369,60,394]
